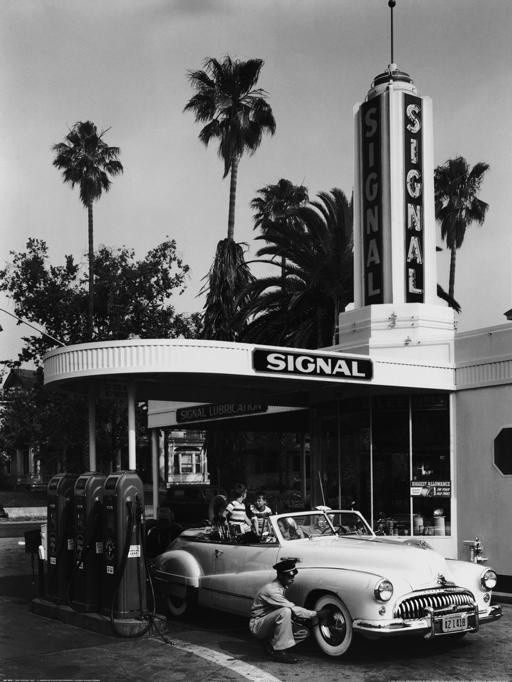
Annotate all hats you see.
[272,559,298,576]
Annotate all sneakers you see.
[266,648,298,663]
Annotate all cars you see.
[151,494,503,660]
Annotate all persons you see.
[248,560,328,664]
[143,507,186,560]
[201,483,273,537]
